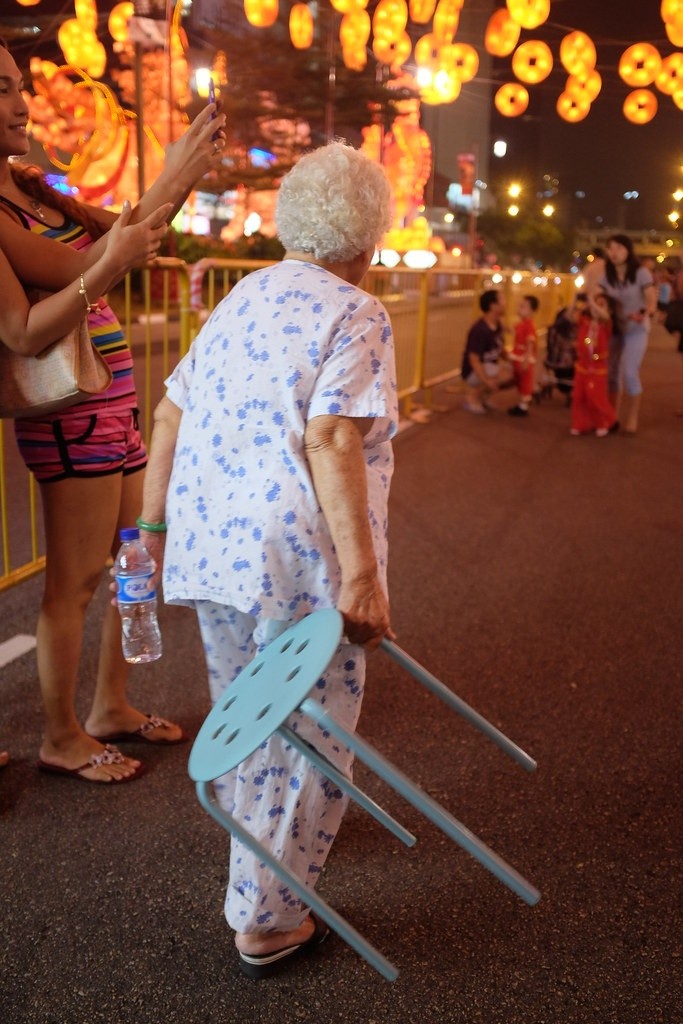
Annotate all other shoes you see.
[507,404,528,417]
[596,428,609,437]
[570,428,584,436]
[462,397,499,415]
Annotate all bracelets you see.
[136,515,167,532]
[78,272,102,315]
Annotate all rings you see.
[212,141,220,152]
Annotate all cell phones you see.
[208,78,220,142]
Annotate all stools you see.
[187,611,543,979]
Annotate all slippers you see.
[106,711,188,745]
[237,903,331,978]
[39,742,147,786]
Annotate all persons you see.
[533,234,683,438]
[0,200,174,359]
[1,42,226,786]
[461,289,528,416]
[108,143,399,976]
[506,295,539,417]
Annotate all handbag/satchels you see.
[0,288,114,419]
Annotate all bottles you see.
[115,528,162,664]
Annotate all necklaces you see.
[0,183,47,220]
